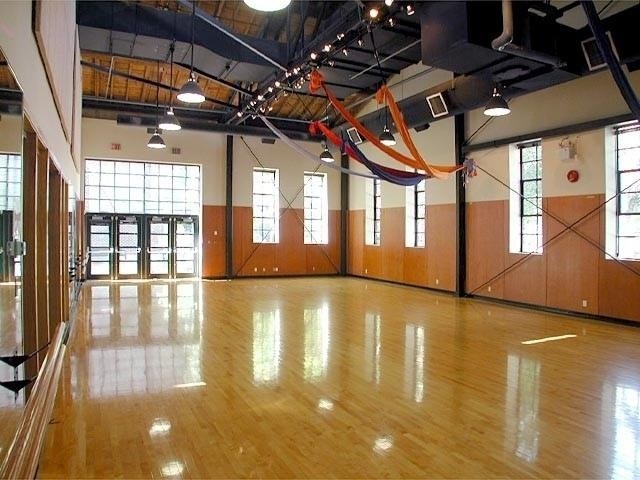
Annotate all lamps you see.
[146,3,205,150]
[319,70,511,164]
